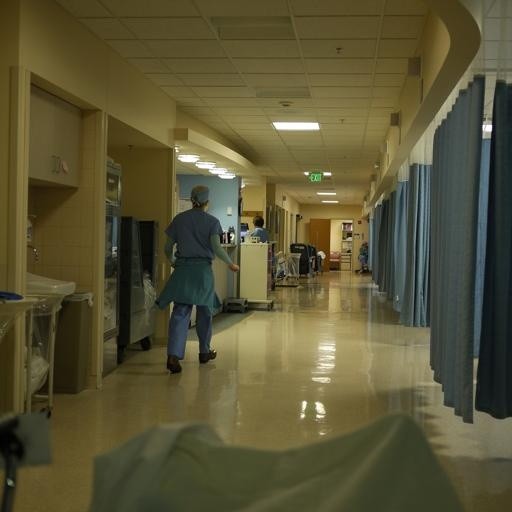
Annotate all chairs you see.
[290,243,322,278]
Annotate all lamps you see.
[176,155,236,180]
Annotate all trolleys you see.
[292,243,325,279]
[117,213,162,364]
[24,294,67,419]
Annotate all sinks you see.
[26,270,76,297]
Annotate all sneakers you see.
[199,347,217,365]
[166,354,182,374]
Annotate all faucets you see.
[28,242,42,262]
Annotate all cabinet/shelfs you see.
[28,82,83,189]
[105,163,124,209]
[338,222,353,271]
[239,242,274,311]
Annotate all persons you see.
[250,215,270,243]
[155,186,239,373]
[359,242,368,273]
